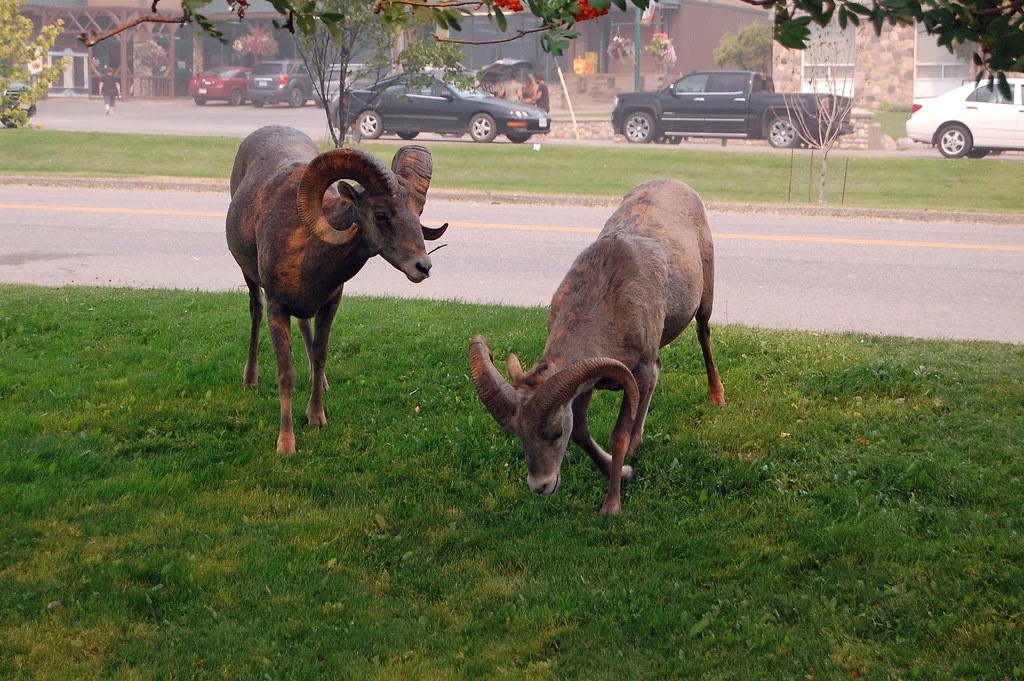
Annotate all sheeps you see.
[225,124,449,456]
[467,175,725,516]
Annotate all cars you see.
[311,63,369,108]
[906,78,1024,158]
[328,70,552,143]
[189,66,252,106]
[0,83,37,128]
[248,60,318,107]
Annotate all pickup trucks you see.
[611,71,854,148]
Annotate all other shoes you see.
[106,110,109,115]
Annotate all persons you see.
[532,75,549,113]
[127,55,133,96]
[522,74,538,103]
[99,67,120,116]
[159,48,171,95]
[494,73,522,102]
